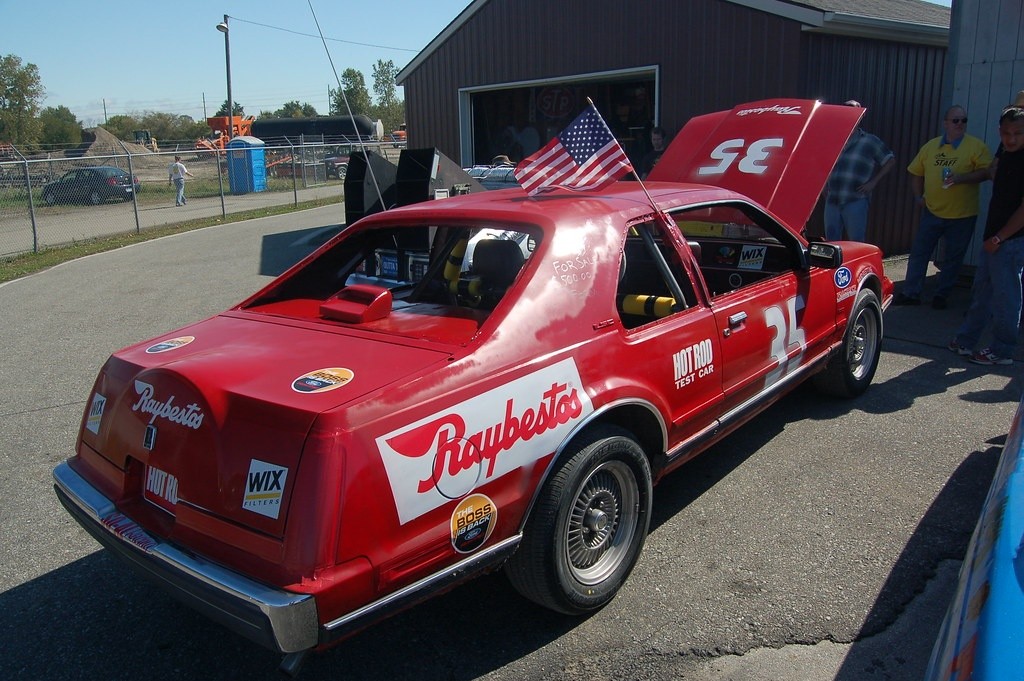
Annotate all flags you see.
[512,104,632,196]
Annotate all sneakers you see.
[947,337,971,355]
[969,346,1014,365]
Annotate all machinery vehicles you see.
[391,124,407,149]
[133,130,159,154]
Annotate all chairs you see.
[473,240,525,310]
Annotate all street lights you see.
[215,21,233,142]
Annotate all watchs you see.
[992,233,1002,245]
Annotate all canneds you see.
[943,166,951,182]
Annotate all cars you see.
[51,182,896,659]
[43,166,140,205]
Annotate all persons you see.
[949,91,1024,364]
[640,126,666,179]
[822,99,897,243]
[169,154,193,206]
[892,103,995,309]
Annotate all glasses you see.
[945,118,968,123]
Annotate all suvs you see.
[319,145,382,180]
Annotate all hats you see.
[843,99,861,107]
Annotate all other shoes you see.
[182,201,186,205]
[890,294,921,306]
[932,295,951,309]
[177,205,182,207]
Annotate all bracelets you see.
[914,195,923,200]
